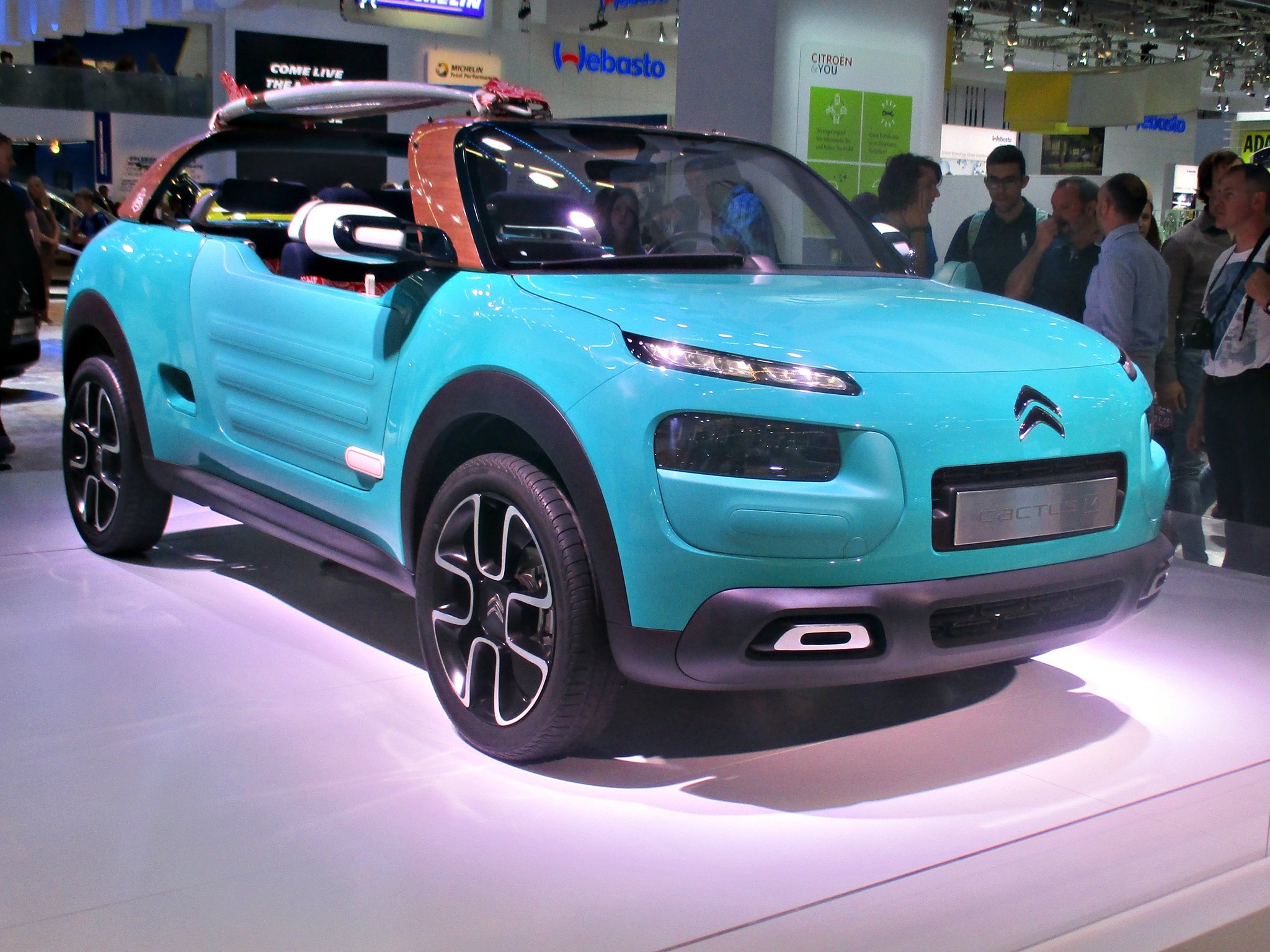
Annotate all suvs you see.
[57,69,1178,766]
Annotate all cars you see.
[165,167,312,221]
[12,181,118,280]
[484,190,603,247]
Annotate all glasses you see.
[983,175,1021,190]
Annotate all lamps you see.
[656,22,666,44]
[623,23,631,40]
[951,0,1270,112]
[518,0,531,19]
[589,7,612,31]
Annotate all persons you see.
[1004,176,1100,325]
[99,185,116,217]
[869,153,942,281]
[1159,151,1245,564]
[1082,172,1171,394]
[25,175,60,326]
[1200,161,1270,574]
[645,158,781,265]
[68,187,108,254]
[0,132,52,472]
[944,144,1051,295]
[600,187,641,256]
[1135,175,1161,250]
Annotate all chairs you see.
[488,184,609,259]
[283,182,399,279]
